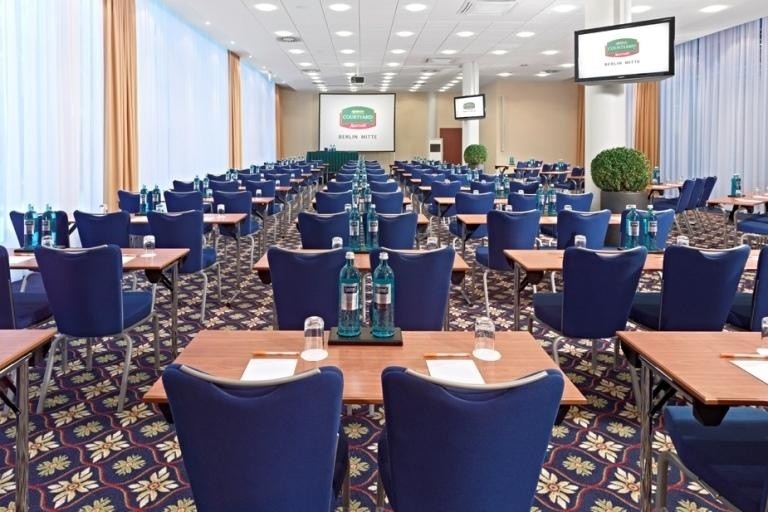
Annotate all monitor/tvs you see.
[454,93,485,119]
[575,16,675,82]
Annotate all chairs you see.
[0,156,767,512]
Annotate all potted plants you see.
[589,146,654,214]
[464,144,488,173]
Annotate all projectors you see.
[351,77,364,83]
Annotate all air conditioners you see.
[428,137,443,164]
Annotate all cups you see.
[761,315,767,351]
[304,316,323,360]
[473,317,494,356]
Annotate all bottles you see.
[336,252,362,337]
[139,184,149,212]
[40,203,57,250]
[150,183,161,211]
[547,183,559,216]
[351,158,372,204]
[528,157,568,171]
[652,165,742,197]
[643,204,658,250]
[347,204,363,251]
[193,157,300,192]
[624,204,640,248]
[372,253,399,337]
[366,204,380,251]
[413,157,511,195]
[535,183,547,216]
[22,202,39,251]
